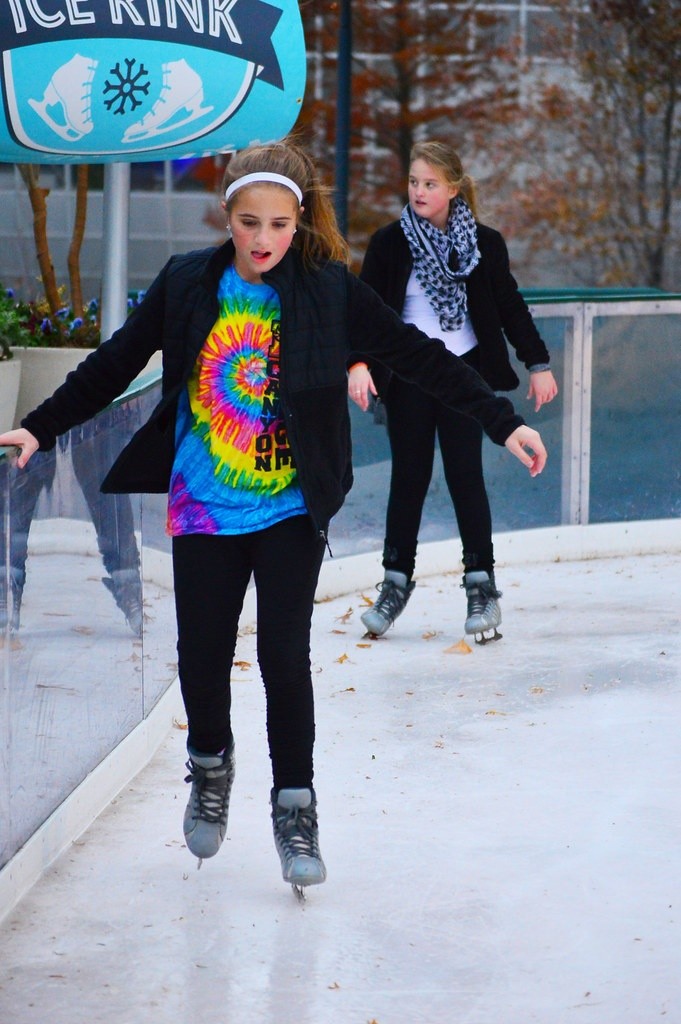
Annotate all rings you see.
[355,390,360,394]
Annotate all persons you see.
[1,134,548,914]
[344,141,559,646]
[1,389,143,636]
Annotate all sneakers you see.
[460,570,502,645]
[362,569,416,639]
[102,569,142,638]
[0,567,25,638]
[271,786,327,905]
[183,734,235,870]
